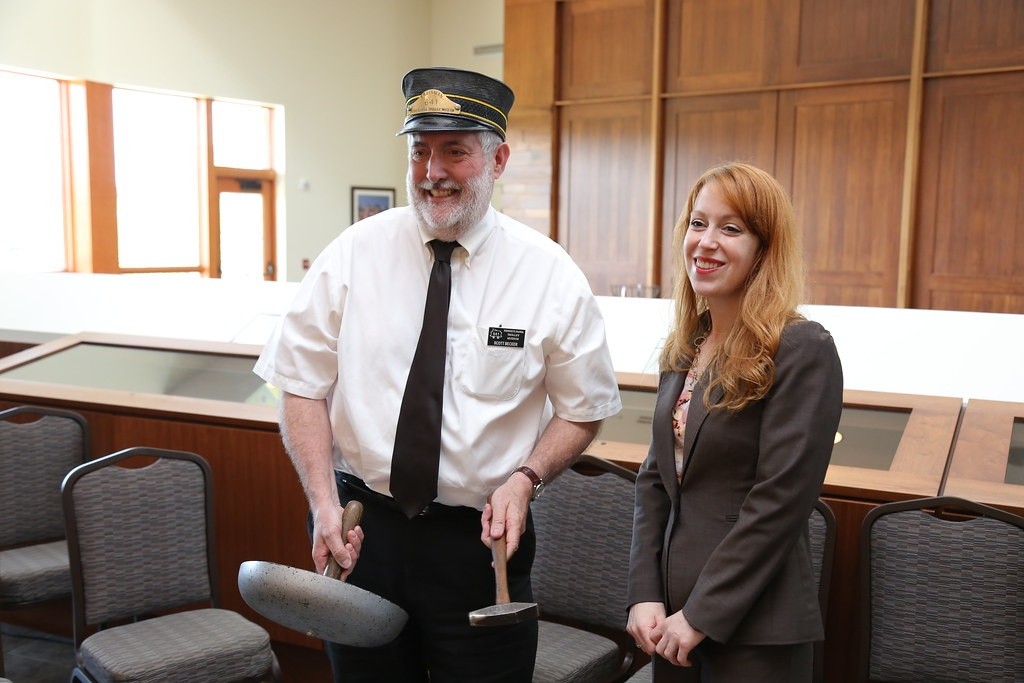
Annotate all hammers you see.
[464,498,542,630]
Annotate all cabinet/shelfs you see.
[500,0,1024,317]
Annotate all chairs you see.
[857,496,1024,683]
[620,495,838,682]
[0,405,91,679]
[531,454,639,683]
[62,446,282,683]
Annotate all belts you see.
[333,470,476,513]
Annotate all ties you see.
[389,239,463,520]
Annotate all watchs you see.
[509,466,546,502]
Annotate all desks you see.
[940,395,1024,527]
[0,331,323,652]
[574,369,966,682]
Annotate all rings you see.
[636,645,641,649]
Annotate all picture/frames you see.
[352,186,396,227]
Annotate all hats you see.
[396,68,515,143]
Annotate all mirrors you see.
[0,0,1024,316]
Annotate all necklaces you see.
[693,324,713,384]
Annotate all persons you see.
[624,161,844,683]
[251,63,624,683]
